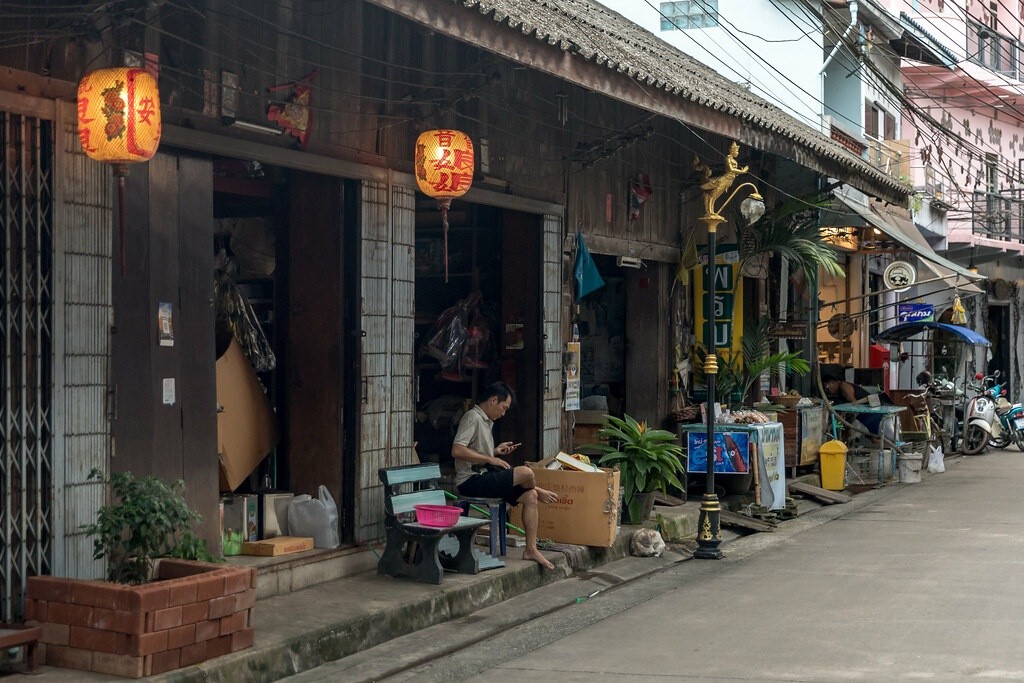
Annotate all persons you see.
[823,375,871,403]
[451,381,559,569]
[724,141,750,177]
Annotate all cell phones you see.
[501,442,522,451]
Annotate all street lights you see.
[692,141,767,558]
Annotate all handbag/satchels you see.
[287,484,340,550]
[927,444,945,474]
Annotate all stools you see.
[453,494,506,558]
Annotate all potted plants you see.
[574,414,687,525]
[694,315,812,494]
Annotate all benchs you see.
[378,461,494,584]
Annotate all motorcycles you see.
[960,370,1007,455]
[975,376,1024,452]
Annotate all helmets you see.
[916,371,932,384]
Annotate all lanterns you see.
[413,129,476,284]
[77,68,162,277]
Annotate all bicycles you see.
[902,381,946,470]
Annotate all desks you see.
[681,422,787,510]
[829,403,908,485]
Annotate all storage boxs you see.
[509,460,619,548]
[239,536,315,555]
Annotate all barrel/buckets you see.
[896,453,923,484]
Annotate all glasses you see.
[825,383,830,392]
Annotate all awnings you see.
[857,206,985,324]
[817,191,989,325]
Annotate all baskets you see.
[413,503,464,527]
[909,393,934,417]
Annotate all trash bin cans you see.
[899,454,923,484]
[819,440,848,490]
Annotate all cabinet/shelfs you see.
[777,407,828,477]
[414,225,485,442]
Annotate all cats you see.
[630,528,666,558]
